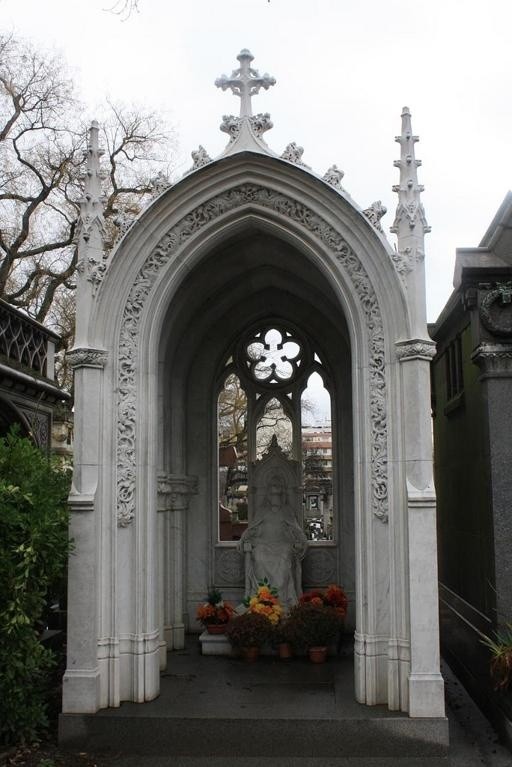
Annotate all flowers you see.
[195,577,350,646]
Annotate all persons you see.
[236,480,308,617]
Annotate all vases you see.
[243,644,327,664]
